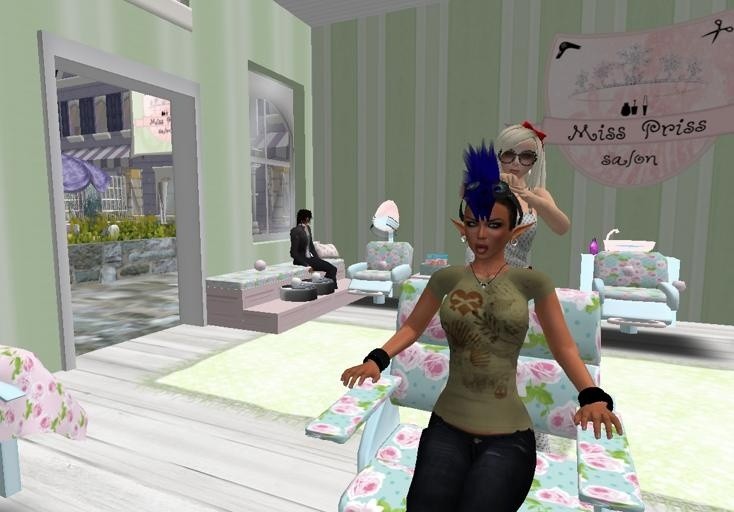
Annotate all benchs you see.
[205,237,365,335]
